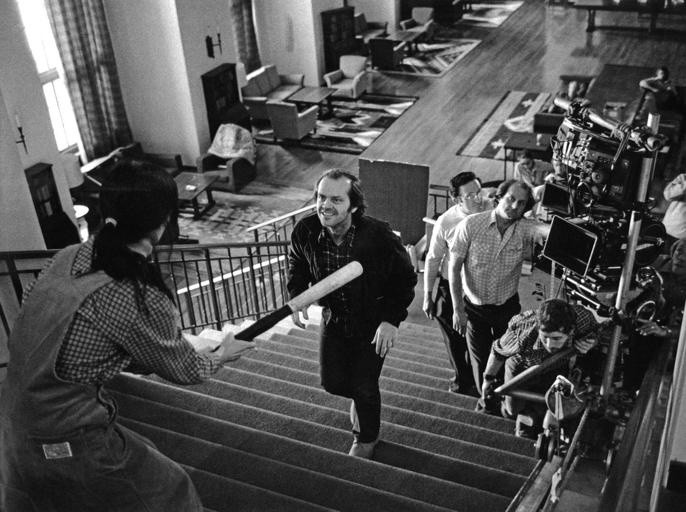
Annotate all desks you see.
[583,63,657,135]
[504,132,555,182]
[573,1,660,36]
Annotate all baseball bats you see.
[212,261,363,353]
[494,329,602,392]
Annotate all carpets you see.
[452,1,527,29]
[365,33,483,79]
[147,175,316,275]
[254,87,419,156]
[454,91,555,162]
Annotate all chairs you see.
[195,124,257,193]
[323,55,369,101]
[369,39,406,71]
[400,7,436,43]
[265,99,320,145]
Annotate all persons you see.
[663,173,686,249]
[0,158,257,512]
[480,297,605,419]
[424,169,564,397]
[446,177,589,398]
[513,154,552,185]
[609,237,686,386]
[285,169,418,461]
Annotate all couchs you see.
[79,143,182,209]
[533,72,597,134]
[351,13,389,56]
[239,65,304,122]
[655,83,685,163]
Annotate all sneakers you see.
[350,398,357,423]
[348,424,382,459]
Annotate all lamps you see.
[205,24,221,57]
[14,113,28,155]
[60,153,84,216]
[237,63,249,88]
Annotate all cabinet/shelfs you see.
[200,62,252,145]
[23,161,79,249]
[320,6,355,74]
